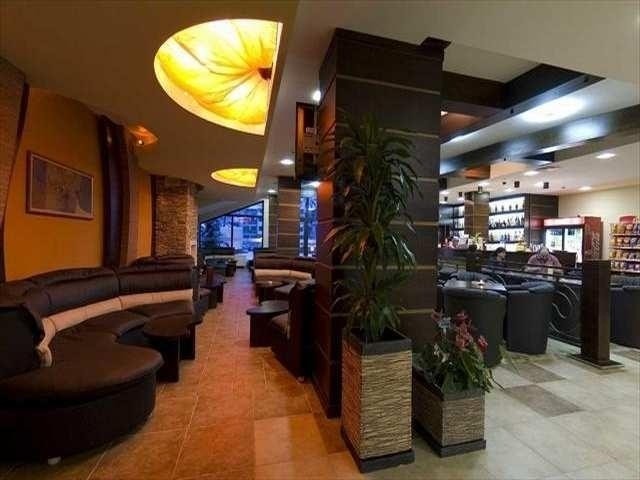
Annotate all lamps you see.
[209,166,261,189]
[150,16,286,138]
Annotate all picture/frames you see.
[23,151,93,219]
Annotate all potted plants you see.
[329,97,424,473]
[413,304,495,458]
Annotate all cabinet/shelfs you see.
[441,203,489,239]
[489,194,557,249]
[604,217,640,274]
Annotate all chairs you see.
[454,270,492,282]
[508,282,558,357]
[610,283,640,348]
[442,284,506,368]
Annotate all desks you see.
[247,299,289,349]
[256,278,283,301]
[145,314,205,384]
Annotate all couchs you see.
[133,252,211,317]
[253,255,314,381]
[1,256,195,467]
[202,244,236,276]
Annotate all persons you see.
[526,247,564,276]
[489,246,512,273]
